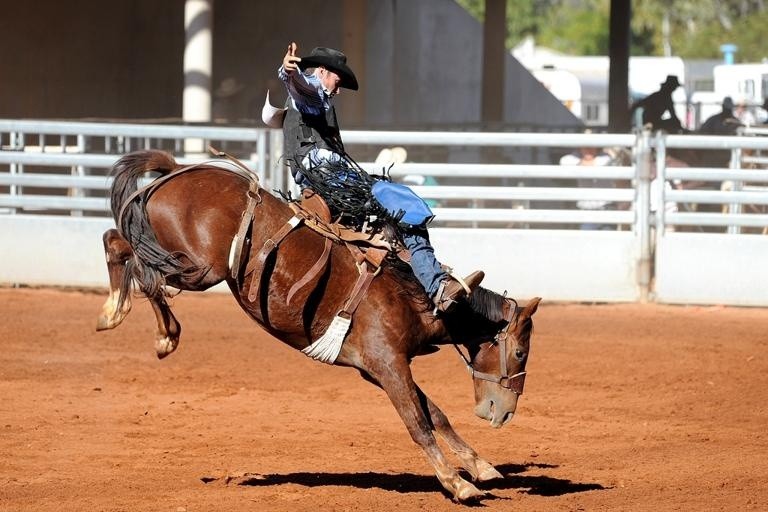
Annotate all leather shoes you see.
[433,271,484,315]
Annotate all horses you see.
[95,149,543,506]
[600,118,768,233]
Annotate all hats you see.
[373,146,407,164]
[295,47,359,91]
[716,97,738,107]
[661,75,682,86]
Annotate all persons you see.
[628,74,697,163]
[699,97,745,170]
[279,41,487,318]
